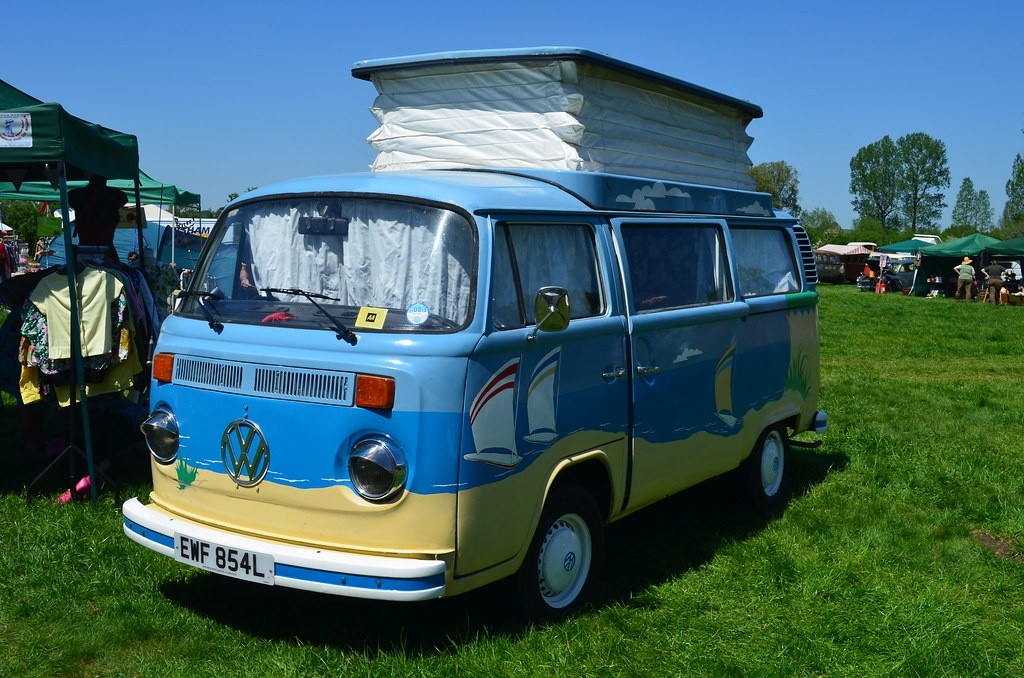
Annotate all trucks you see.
[1000,262,1022,282]
[816,242,877,285]
[140,219,241,269]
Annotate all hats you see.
[962,257,973,265]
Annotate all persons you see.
[240,238,259,287]
[37,237,44,258]
[863,263,875,289]
[954,257,977,300]
[981,258,1005,305]
[68,175,128,261]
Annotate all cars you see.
[857,257,916,293]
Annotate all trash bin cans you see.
[875,282,885,294]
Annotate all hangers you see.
[57,244,108,273]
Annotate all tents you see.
[0,79,147,502]
[0,167,203,269]
[914,234,1002,298]
[878,239,936,280]
[984,237,1024,256]
[819,244,871,255]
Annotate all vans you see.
[120,48,829,621]
[891,255,916,272]
